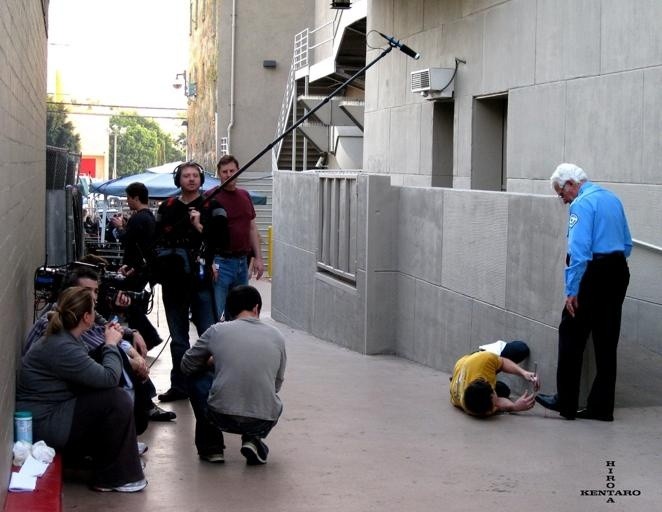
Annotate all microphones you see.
[379,32,420,60]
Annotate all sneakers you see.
[240,437,269,465]
[137,440,149,454]
[147,403,177,422]
[95,475,150,492]
[199,447,226,464]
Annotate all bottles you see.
[14,411,33,445]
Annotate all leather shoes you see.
[534,392,577,420]
[578,407,615,422]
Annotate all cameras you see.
[35,260,150,317]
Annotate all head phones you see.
[172,162,206,187]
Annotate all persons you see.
[202,154,265,321]
[15,285,149,493]
[84,212,128,266]
[36,303,177,422]
[156,163,228,403]
[448,340,541,419]
[110,182,163,350]
[534,162,633,422]
[179,284,287,463]
[22,267,150,457]
